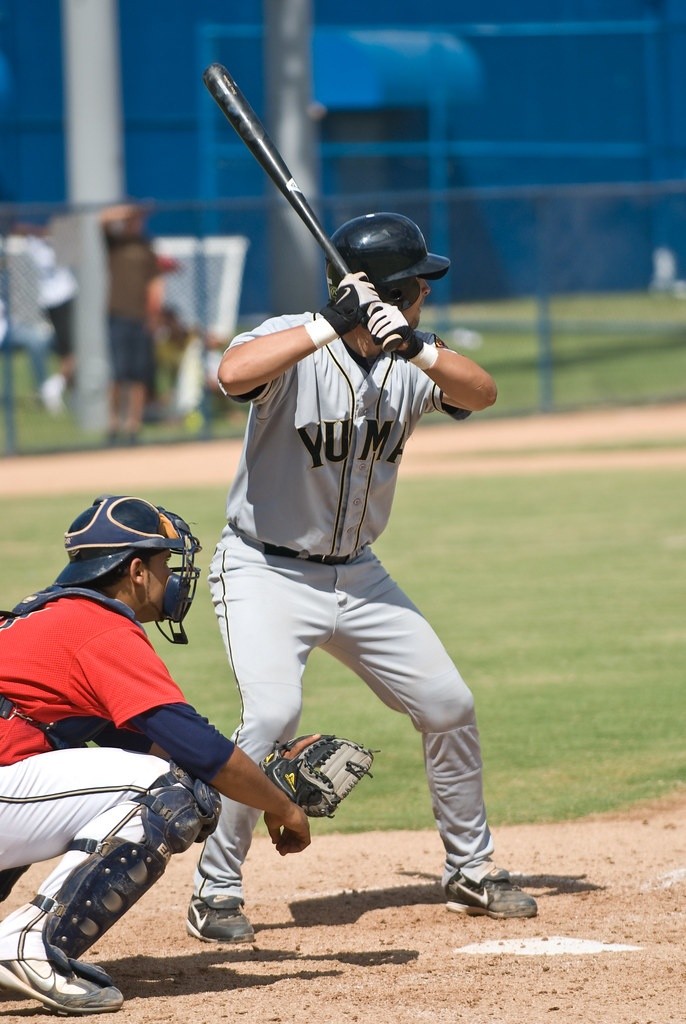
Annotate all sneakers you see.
[186,893,255,943]
[444,866,537,919]
[0,960,125,1016]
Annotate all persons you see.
[0,203,233,441]
[181,214,543,946]
[0,496,310,1016]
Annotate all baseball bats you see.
[203,62,406,356]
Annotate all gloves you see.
[360,301,424,363]
[319,271,384,339]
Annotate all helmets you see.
[325,212,451,312]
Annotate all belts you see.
[263,542,369,566]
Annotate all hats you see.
[52,493,169,587]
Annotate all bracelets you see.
[302,318,337,346]
[410,344,439,371]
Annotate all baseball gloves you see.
[261,732,377,817]
